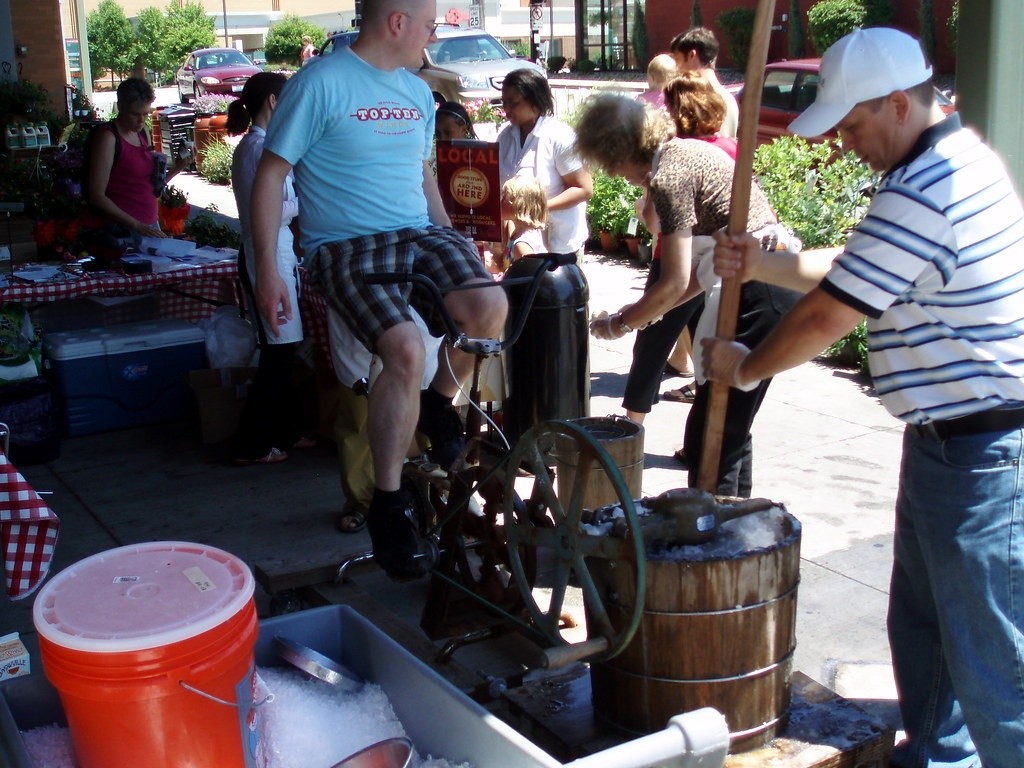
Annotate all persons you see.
[701,27,1024,767]
[302,37,314,66]
[92,77,170,241]
[228,75,317,464]
[255,0,509,574]
[575,92,799,499]
[624,27,740,460]
[439,66,594,405]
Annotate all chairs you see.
[762,85,784,106]
[801,82,817,110]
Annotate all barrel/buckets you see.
[555,417,644,518]
[33,540,274,768]
[583,496,802,753]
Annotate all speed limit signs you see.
[469,3,480,28]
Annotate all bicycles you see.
[352,247,647,666]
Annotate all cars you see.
[312,31,363,58]
[252,52,268,66]
[721,57,958,199]
[402,27,548,121]
[175,48,266,104]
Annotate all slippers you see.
[336,504,371,532]
[662,361,682,375]
[663,386,696,403]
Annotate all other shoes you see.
[260,450,287,462]
[417,388,468,464]
[367,502,426,584]
[292,437,317,448]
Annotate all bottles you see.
[5,121,51,148]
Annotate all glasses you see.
[389,11,439,37]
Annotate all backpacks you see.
[79,121,147,183]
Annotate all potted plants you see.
[805,182,871,367]
[197,131,233,185]
[6,157,100,247]
[65,83,92,118]
[157,185,190,234]
[585,175,654,268]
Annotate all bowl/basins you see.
[331,737,413,768]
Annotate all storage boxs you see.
[41,317,210,435]
[189,368,262,447]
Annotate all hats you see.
[787,27,933,137]
